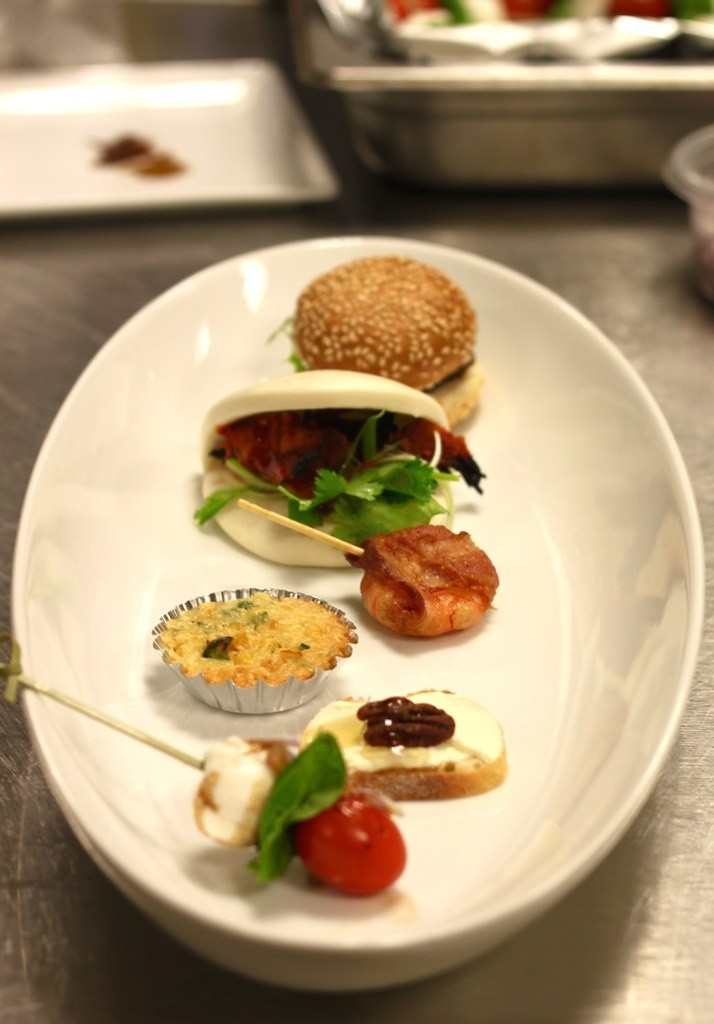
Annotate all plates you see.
[0,55,339,221]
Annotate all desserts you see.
[152,589,359,715]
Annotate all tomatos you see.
[292,791,407,897]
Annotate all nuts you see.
[357,696,457,749]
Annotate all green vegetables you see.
[245,734,348,882]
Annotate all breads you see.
[298,688,513,802]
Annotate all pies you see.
[193,368,489,569]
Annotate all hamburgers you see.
[289,254,480,431]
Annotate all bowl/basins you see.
[659,123,714,301]
[9,236,707,994]
[282,1,713,192]
